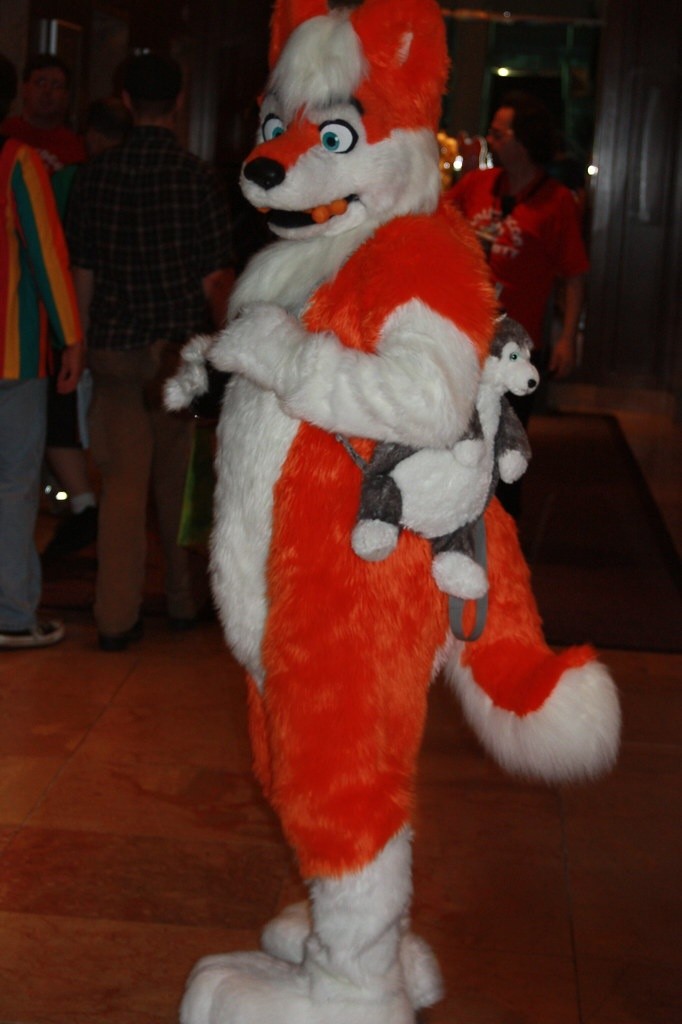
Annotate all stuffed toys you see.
[351,317,539,598]
[166,0,620,1024]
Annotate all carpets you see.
[35,411,682,652]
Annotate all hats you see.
[125,55,182,97]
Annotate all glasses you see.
[31,78,65,89]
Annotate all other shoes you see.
[0,619,65,647]
[44,505,98,557]
[100,620,144,649]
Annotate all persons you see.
[446,102,589,521]
[0,53,265,651]
